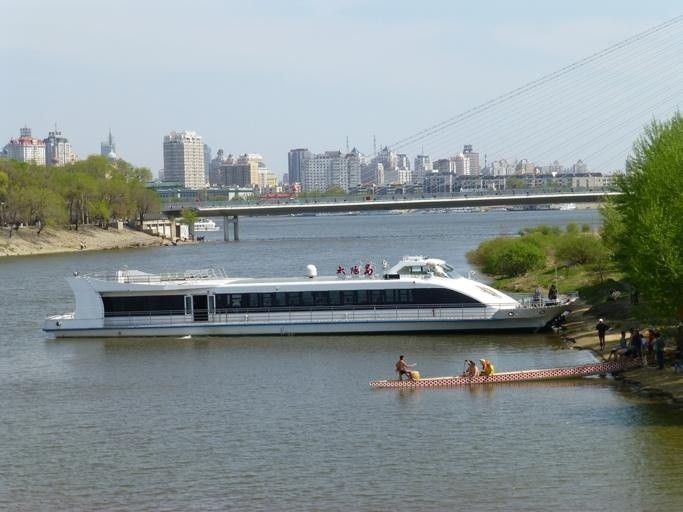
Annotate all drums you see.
[410,370,420,381]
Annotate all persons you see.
[620,327,665,371]
[631,283,638,304]
[533,283,544,300]
[548,284,557,299]
[464,362,479,379]
[596,317,610,351]
[481,360,494,376]
[395,354,417,381]
[479,359,487,375]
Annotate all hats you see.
[470,360,475,365]
[480,359,485,362]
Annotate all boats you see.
[369,360,641,388]
[193,218,219,233]
[43,255,577,334]
[508,204,561,211]
